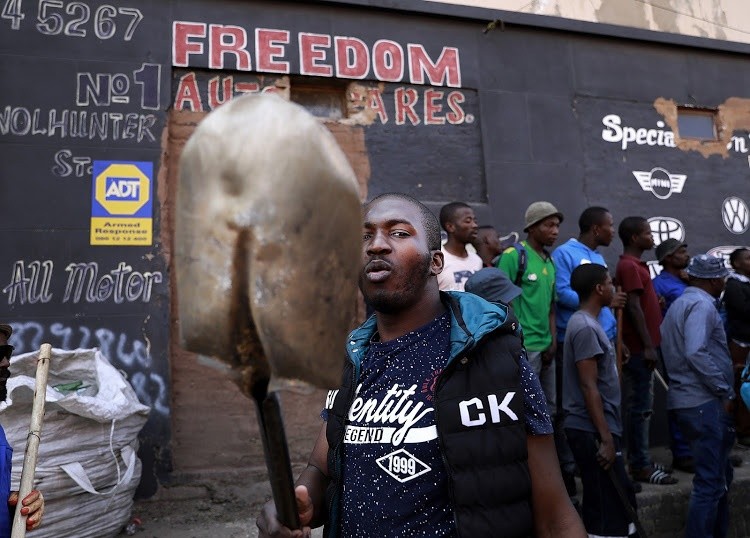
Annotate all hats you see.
[464,267,524,304]
[656,238,688,265]
[687,254,730,279]
[0,324,12,340]
[522,200,565,233]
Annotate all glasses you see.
[0,345,14,362]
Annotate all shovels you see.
[171,93,362,529]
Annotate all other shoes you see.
[730,455,743,467]
[673,455,694,473]
[738,436,750,446]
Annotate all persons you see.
[563,264,639,538]
[254,191,588,538]
[0,324,43,538]
[652,238,691,315]
[436,202,483,292]
[615,217,678,484]
[658,254,739,538]
[497,201,579,505]
[471,223,503,268]
[724,248,750,450]
[549,205,642,493]
[464,267,523,305]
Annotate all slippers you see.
[629,462,680,485]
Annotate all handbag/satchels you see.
[0,348,152,538]
